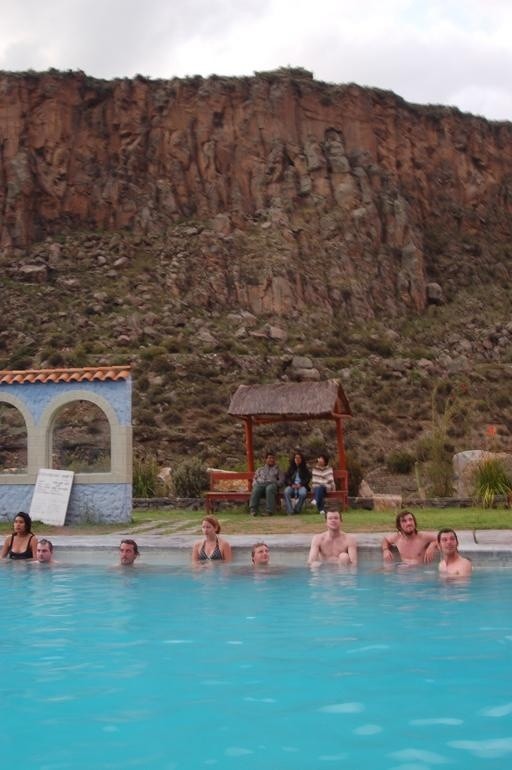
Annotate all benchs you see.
[203,468,350,514]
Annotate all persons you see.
[310,454,336,515]
[382,511,438,563]
[437,528,472,577]
[0,512,38,561]
[251,543,270,565]
[248,453,285,517]
[108,540,139,569]
[308,508,357,565]
[192,516,232,562]
[283,453,312,516]
[31,539,58,564]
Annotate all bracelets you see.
[383,548,389,551]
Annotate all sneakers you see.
[319,509,326,515]
[260,510,273,516]
[250,509,257,516]
[310,498,318,506]
[287,509,299,515]
